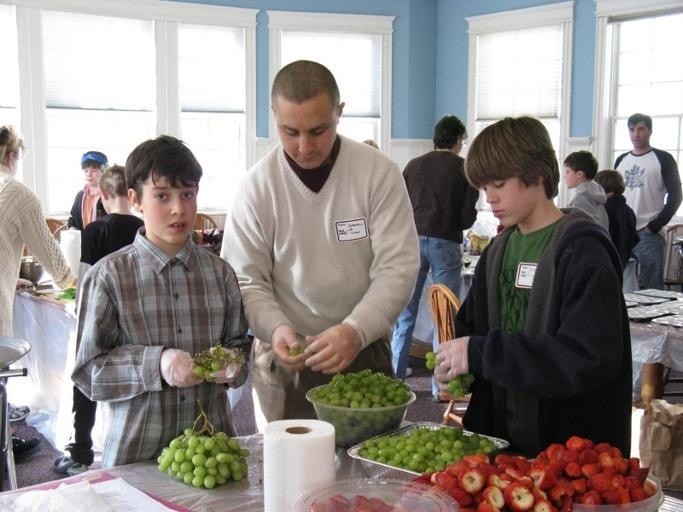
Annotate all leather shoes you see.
[11,436,42,462]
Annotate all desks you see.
[0,376,683,512]
[623,288,683,404]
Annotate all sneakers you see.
[8,401,31,423]
[53,456,88,477]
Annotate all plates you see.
[6,435,38,455]
[6,403,29,420]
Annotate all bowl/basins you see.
[291,475,460,511]
[19,257,45,291]
[572,470,665,511]
[303,382,417,448]
[462,257,471,267]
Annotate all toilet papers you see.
[60,227,82,279]
[264,420,336,512]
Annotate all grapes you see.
[288,347,305,356]
[309,368,410,448]
[359,427,499,475]
[425,352,475,398]
[156,397,248,490]
[191,350,227,383]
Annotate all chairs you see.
[428,283,472,426]
[194,214,217,228]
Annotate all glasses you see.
[462,139,467,147]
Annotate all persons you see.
[563,151,610,234]
[68,151,109,234]
[612,114,683,291]
[71,137,251,466]
[594,170,639,271]
[221,60,421,433]
[388,115,479,402]
[0,127,76,458]
[435,115,629,467]
[53,165,144,478]
